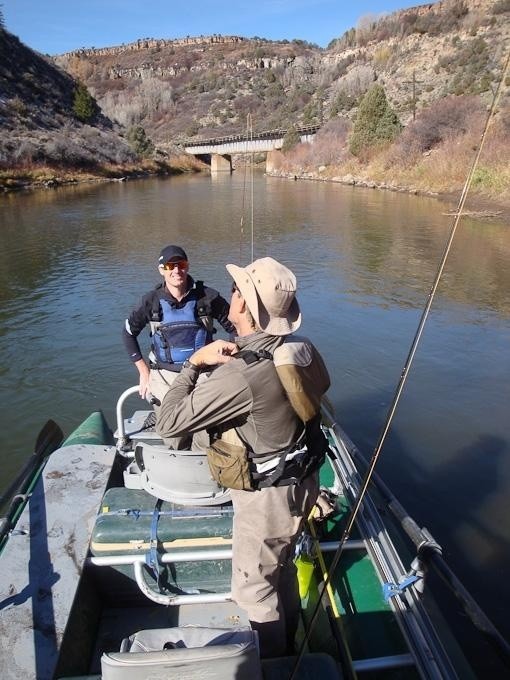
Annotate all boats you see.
[0,401,508,678]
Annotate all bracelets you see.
[182,359,200,372]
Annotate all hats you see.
[226,257,301,337]
[159,245,187,265]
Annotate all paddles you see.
[0,418,64,555]
[316,400,508,657]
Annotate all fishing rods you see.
[288,48,508,679]
[238,113,255,265]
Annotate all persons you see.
[121,245,237,451]
[155,256,331,660]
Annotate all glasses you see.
[231,281,239,293]
[164,263,187,270]
